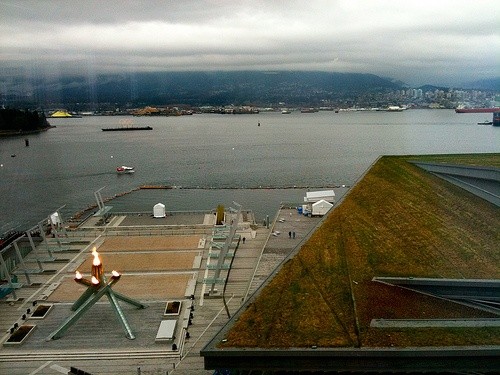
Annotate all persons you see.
[289,231,291,238]
[293,232,295,239]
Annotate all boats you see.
[117,165,135,174]
